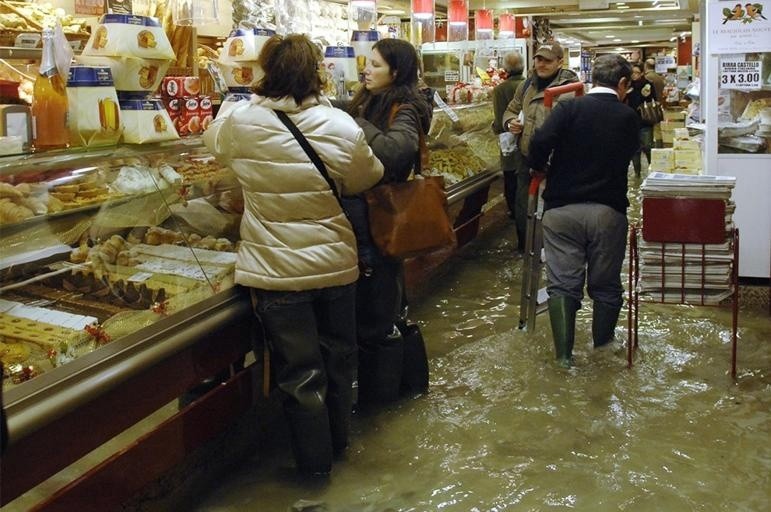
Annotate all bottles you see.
[30,28,68,153]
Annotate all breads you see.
[176,154,229,196]
[1,3,88,34]
[0,309,92,380]
[13,227,243,311]
[0,151,144,229]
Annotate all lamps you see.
[410,1,433,21]
[498,9,514,36]
[447,1,468,24]
[475,0,494,32]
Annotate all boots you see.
[632,152,641,180]
[647,150,651,164]
[591,297,624,348]
[547,294,582,360]
[316,293,359,460]
[365,321,407,403]
[515,221,525,254]
[257,299,333,476]
[403,321,430,391]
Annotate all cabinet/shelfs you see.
[0,134,267,512]
[627,223,740,378]
[0,24,220,105]
[406,99,504,301]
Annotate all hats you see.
[532,41,564,62]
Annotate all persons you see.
[203,31,385,489]
[642,59,664,92]
[493,51,525,219]
[329,39,432,402]
[625,65,657,186]
[502,42,582,256]
[526,52,642,360]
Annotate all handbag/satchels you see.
[341,193,378,278]
[364,174,457,260]
[497,108,524,159]
[636,101,665,128]
